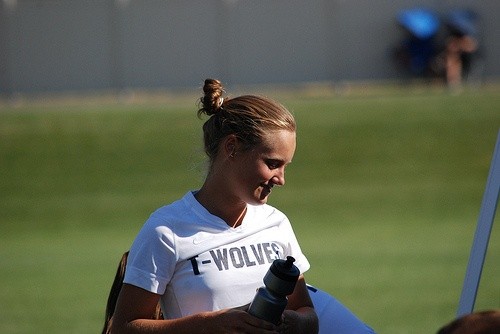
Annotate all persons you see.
[108,79,322,334]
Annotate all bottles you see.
[247,256,300,330]
